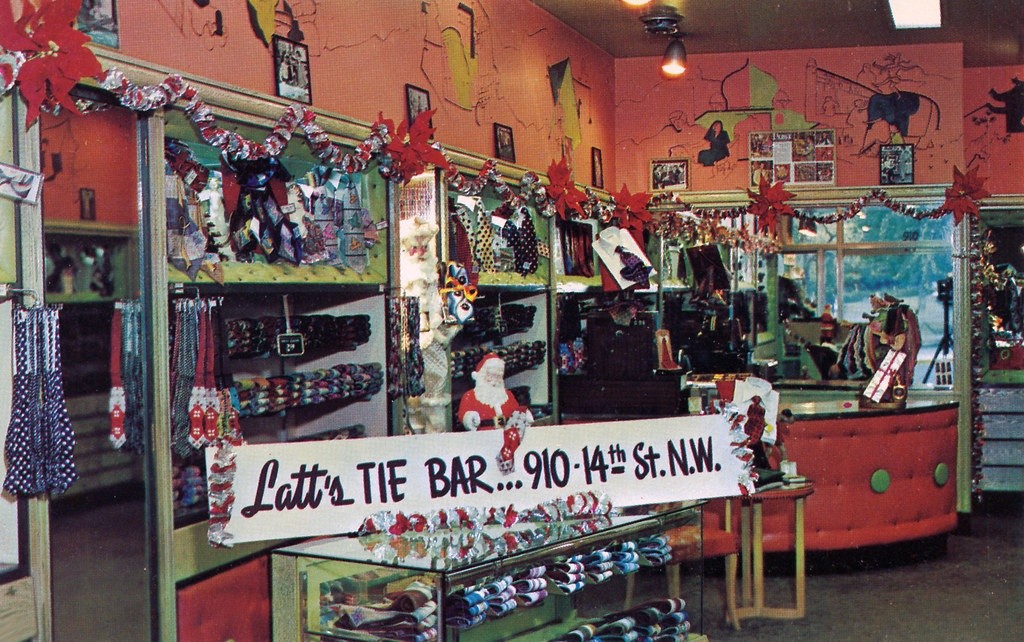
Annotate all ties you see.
[744,396,766,447]
[448,195,550,287]
[662,338,677,369]
[615,246,652,289]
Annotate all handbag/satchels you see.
[702,316,741,352]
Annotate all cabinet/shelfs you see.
[450,283,551,429]
[166,280,385,532]
[42,221,138,502]
[266,507,704,641]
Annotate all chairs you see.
[619,503,740,635]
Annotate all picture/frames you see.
[880,143,914,185]
[493,122,516,164]
[649,157,691,193]
[272,34,312,105]
[72,0,120,53]
[747,128,837,189]
[405,83,433,139]
[591,146,603,189]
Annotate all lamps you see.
[638,5,689,76]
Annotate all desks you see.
[724,480,815,630]
